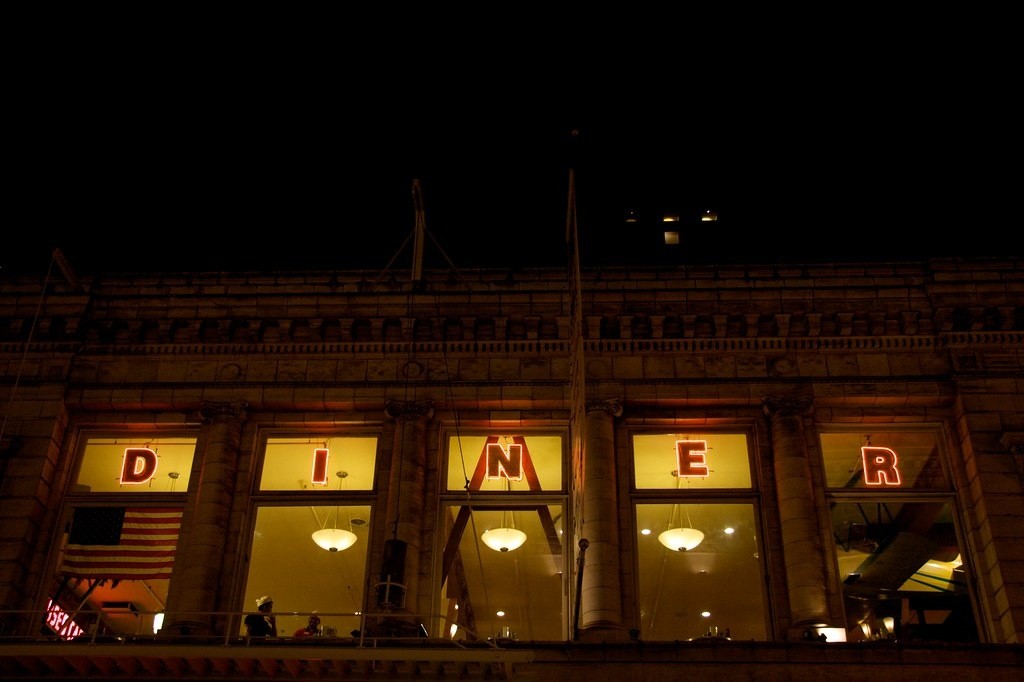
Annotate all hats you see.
[308,615,320,625]
[256,596,273,608]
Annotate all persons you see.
[293,615,321,637]
[245,596,277,641]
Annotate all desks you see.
[875,592,968,640]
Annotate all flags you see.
[60,505,183,579]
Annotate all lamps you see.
[659,469,703,552]
[482,470,527,553]
[858,617,894,640]
[312,472,358,552]
